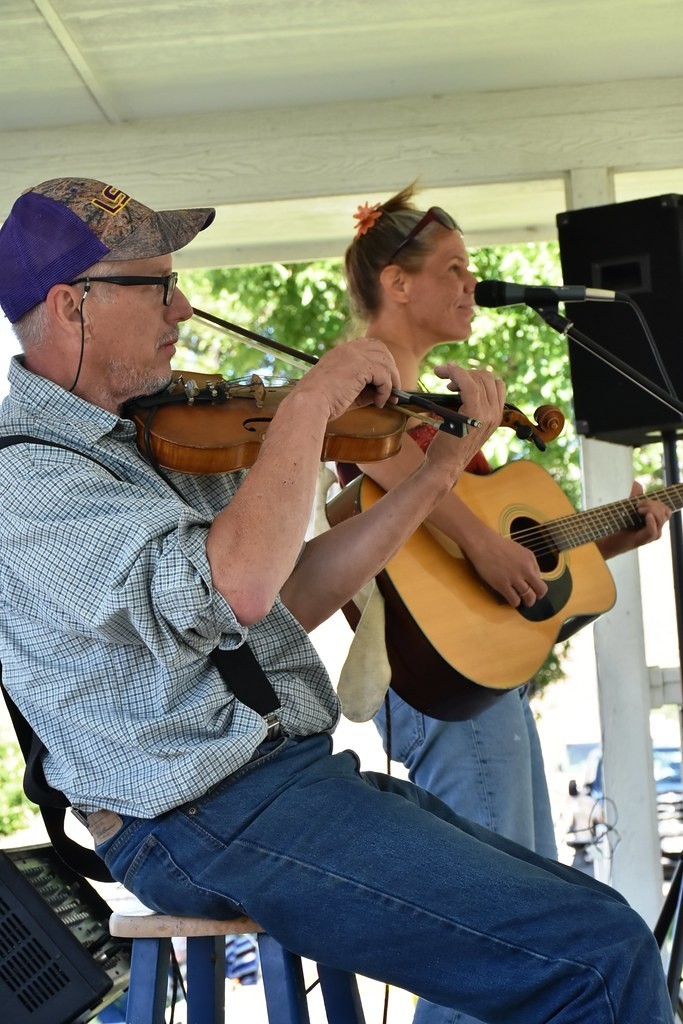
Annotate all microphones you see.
[474,279,628,308]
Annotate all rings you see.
[521,586,531,596]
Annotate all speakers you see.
[556,192,683,448]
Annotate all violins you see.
[131,368,568,476]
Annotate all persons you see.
[1,176,681,1024]
[326,179,673,1024]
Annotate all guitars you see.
[350,457,683,729]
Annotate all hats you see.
[1,178,215,323]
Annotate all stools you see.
[109,907,367,1024]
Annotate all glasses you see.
[43,272,177,308]
[387,206,465,272]
